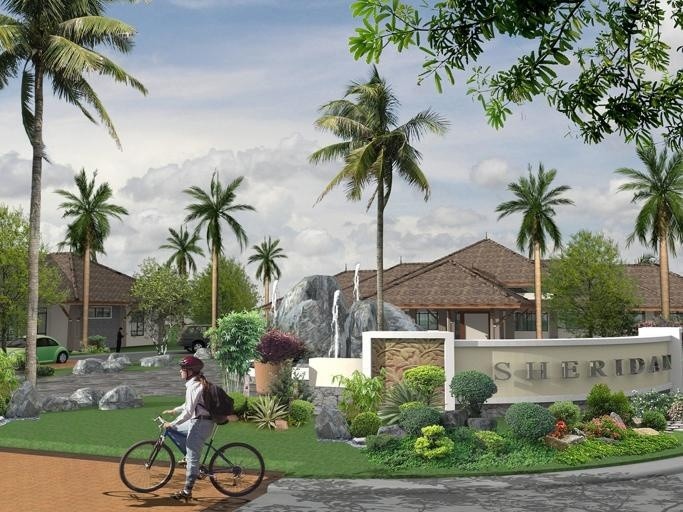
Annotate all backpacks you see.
[198,375,235,416]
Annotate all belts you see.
[192,415,212,420]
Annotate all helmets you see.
[179,356,203,373]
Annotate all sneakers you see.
[178,457,187,464]
[170,488,192,500]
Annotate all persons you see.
[115,327,124,351]
[160,355,215,501]
